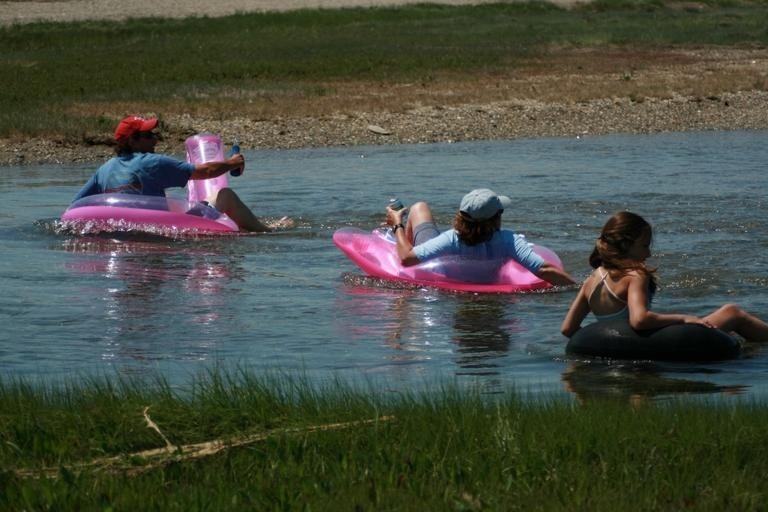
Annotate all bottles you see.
[228,145,242,177]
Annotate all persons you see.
[379,187,578,288]
[71,116,276,232]
[559,211,768,346]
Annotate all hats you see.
[459,187,511,222]
[113,116,158,145]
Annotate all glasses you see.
[138,132,164,143]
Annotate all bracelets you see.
[393,224,403,233]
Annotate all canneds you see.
[389,197,408,224]
